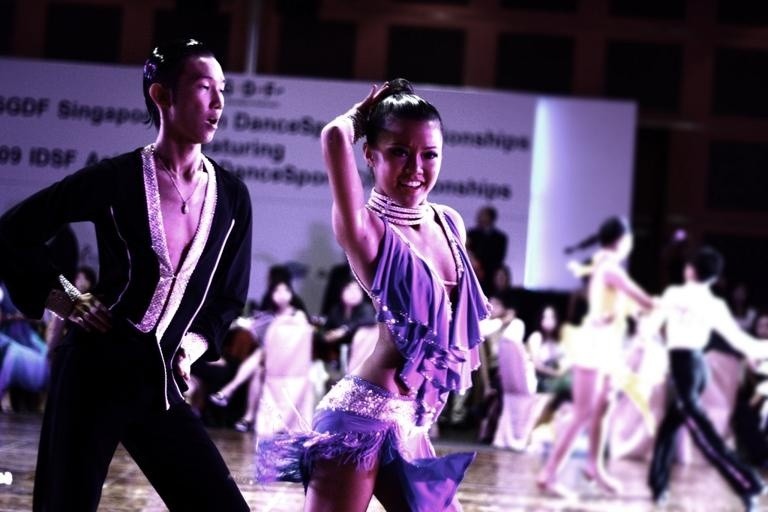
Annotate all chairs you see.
[494,338,559,448]
[260,316,322,435]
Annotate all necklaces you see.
[152,147,204,215]
[368,186,430,226]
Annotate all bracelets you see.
[342,107,365,145]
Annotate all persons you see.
[180,266,378,431]
[0,36,254,512]
[254,77,496,512]
[1,261,98,418]
